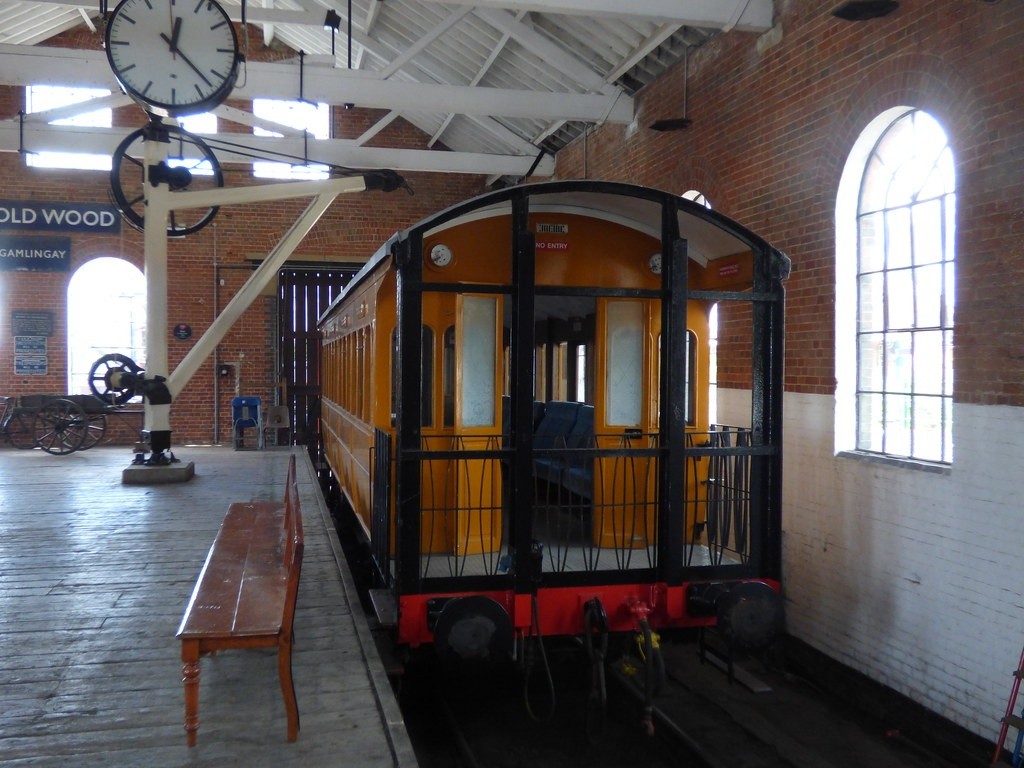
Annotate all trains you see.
[314,177,791,739]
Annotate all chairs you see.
[233,396,265,450]
[265,406,291,446]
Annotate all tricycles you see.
[0,393,117,455]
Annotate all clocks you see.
[103,0,241,118]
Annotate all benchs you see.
[172,455,303,749]
